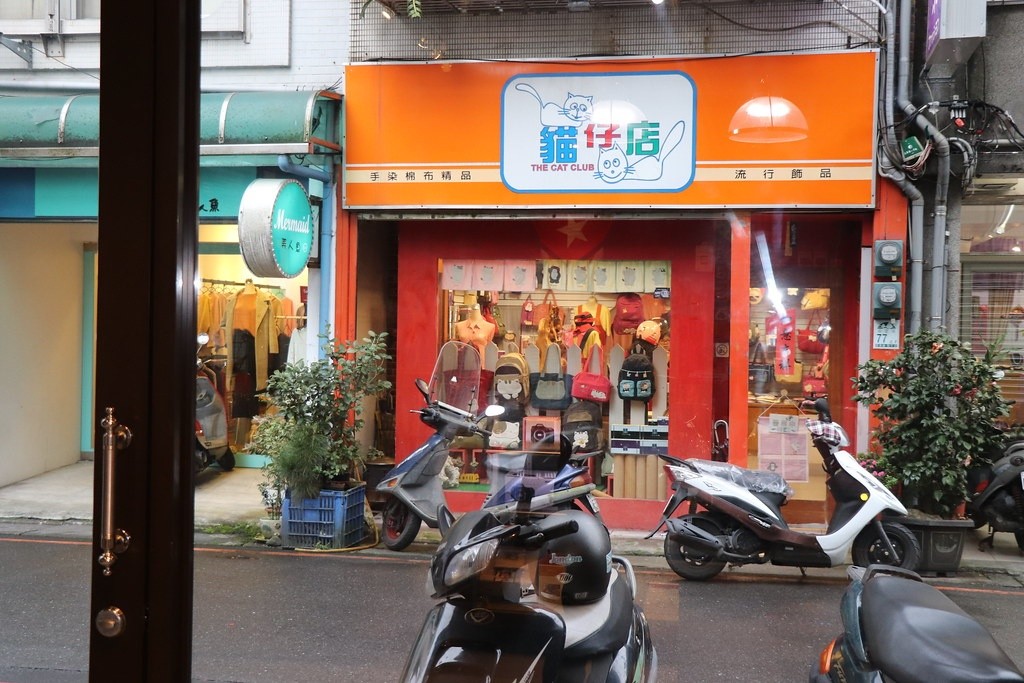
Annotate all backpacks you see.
[619,340,657,398]
[519,294,535,327]
[561,400,604,452]
[492,353,529,408]
[610,292,648,333]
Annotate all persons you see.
[214,278,278,417]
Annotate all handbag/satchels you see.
[529,343,574,411]
[774,352,804,386]
[797,308,830,356]
[572,343,617,404]
[749,343,776,389]
[798,290,830,311]
[804,361,830,399]
[533,289,567,330]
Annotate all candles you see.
[853,324,1014,571]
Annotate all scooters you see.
[642,397,922,583]
[806,562,1022,683]
[397,434,657,683]
[192,331,236,475]
[375,341,605,552]
[965,439,1024,555]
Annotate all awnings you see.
[0,87,341,168]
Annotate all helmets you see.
[653,317,669,339]
[525,510,614,606]
[637,320,660,351]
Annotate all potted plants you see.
[261,330,393,549]
